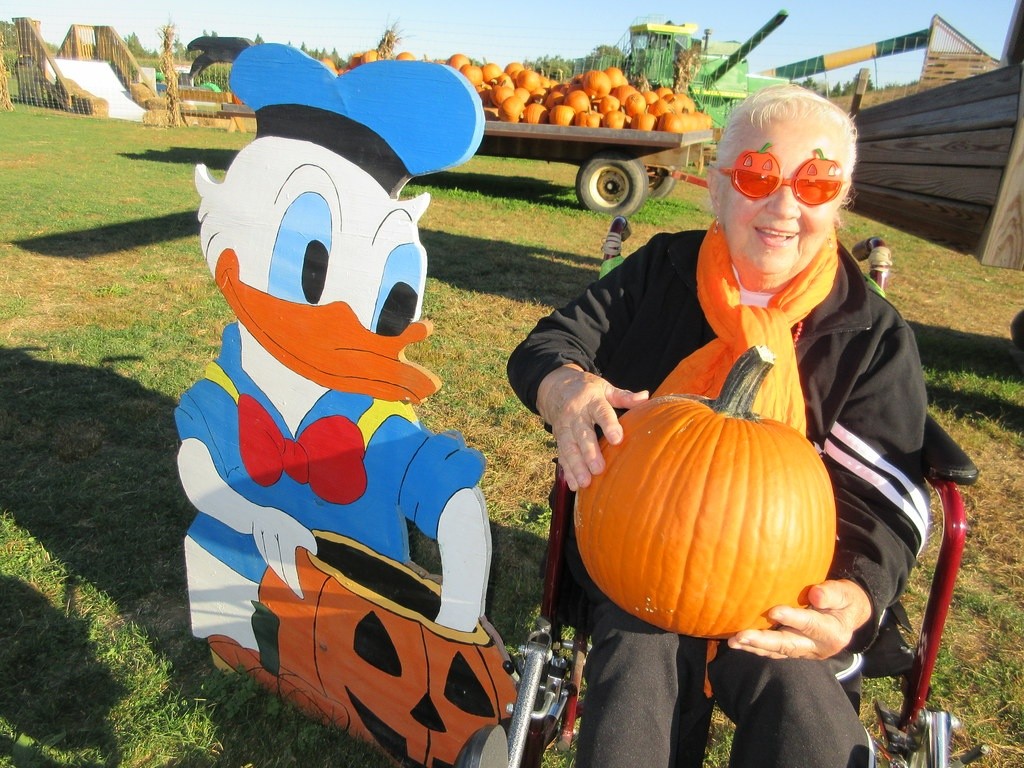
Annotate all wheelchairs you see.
[506,216,990,768]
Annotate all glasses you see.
[718,143,849,206]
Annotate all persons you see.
[505,82,933,768]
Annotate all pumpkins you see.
[576,345,837,641]
[320,52,711,133]
